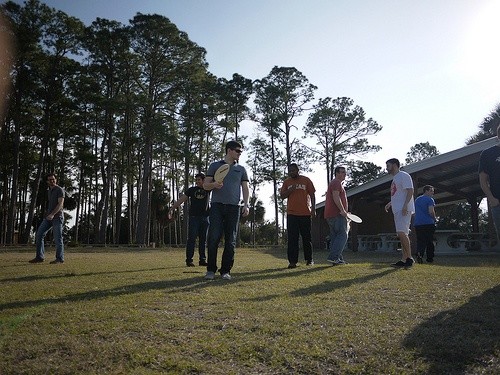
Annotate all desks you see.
[325,230,484,252]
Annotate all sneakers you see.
[390,260,404,267]
[328,258,340,264]
[332,261,345,264]
[205,271,215,279]
[222,272,232,279]
[405,257,414,267]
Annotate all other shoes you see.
[417,257,424,263]
[288,262,299,268]
[427,257,433,262]
[50,258,64,264]
[186,262,195,267]
[28,258,45,263]
[305,260,314,266]
[199,261,208,266]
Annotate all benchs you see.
[386,240,401,249]
[361,241,381,249]
[457,239,496,248]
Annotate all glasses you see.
[231,149,242,154]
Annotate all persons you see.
[384,159,416,268]
[279,163,317,270]
[412,184,440,264]
[203,141,250,281]
[478,123,500,245]
[167,173,213,268]
[29,172,66,264]
[324,166,351,265]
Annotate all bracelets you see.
[433,216,437,219]
